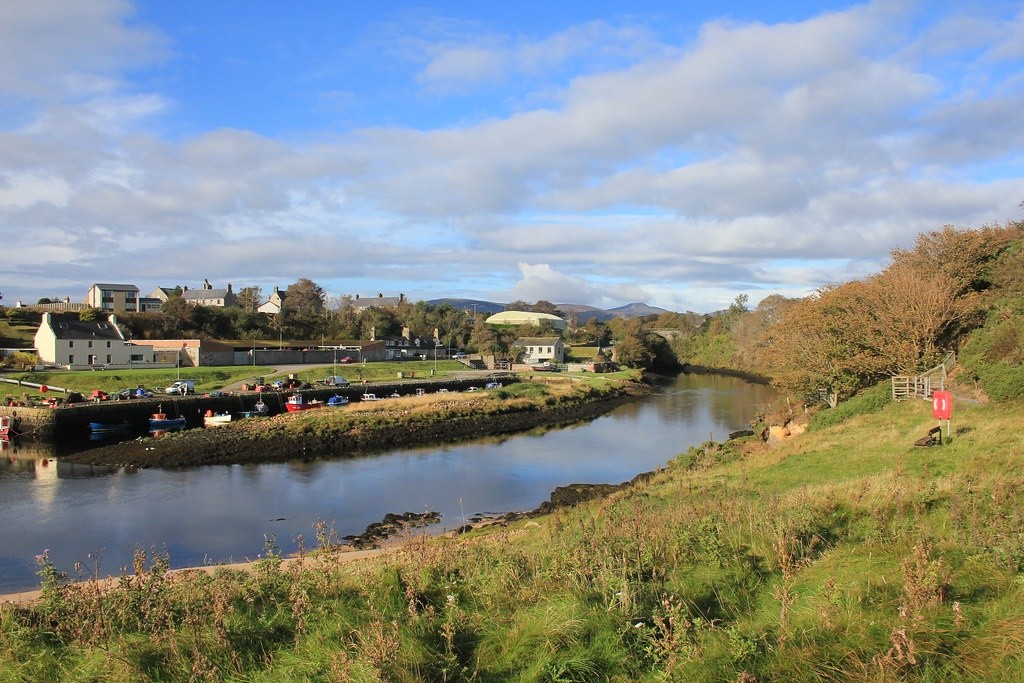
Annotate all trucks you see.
[165,379,195,396]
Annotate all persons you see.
[411,371,415,378]
[180,386,184,397]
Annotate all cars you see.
[326,376,350,388]
[452,352,466,360]
[341,356,357,364]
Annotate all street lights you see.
[435,344,443,370]
[129,329,133,370]
[254,333,256,366]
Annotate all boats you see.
[90,421,132,434]
[391,393,401,399]
[416,388,427,397]
[361,394,381,402]
[236,393,268,418]
[485,383,502,389]
[438,389,448,394]
[147,404,187,433]
[203,410,231,422]
[467,387,478,392]
[325,394,348,406]
[532,361,558,372]
[284,393,325,412]
[0,415,22,443]
